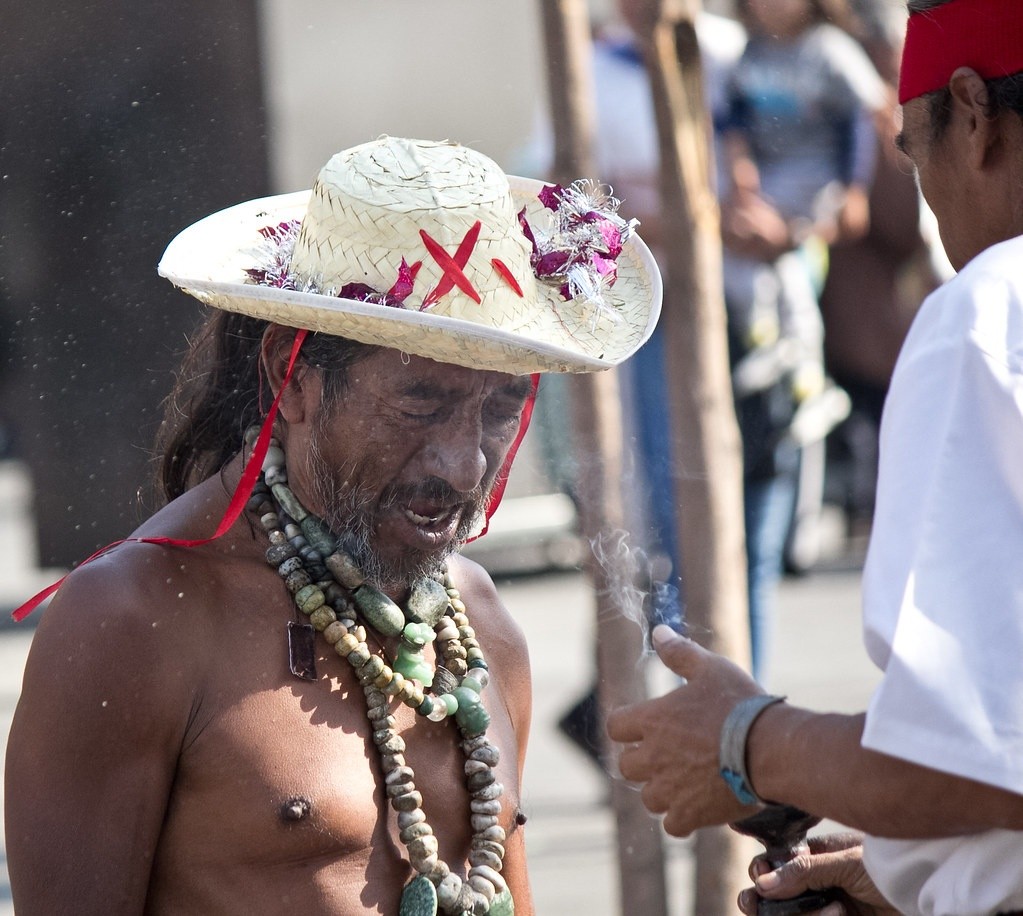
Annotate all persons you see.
[607,0,1023,916]
[530,1,944,688]
[0,135,665,916]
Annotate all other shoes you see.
[561,692,603,760]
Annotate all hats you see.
[157,133,664,374]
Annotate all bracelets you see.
[720,693,788,812]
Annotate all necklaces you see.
[244,425,517,916]
